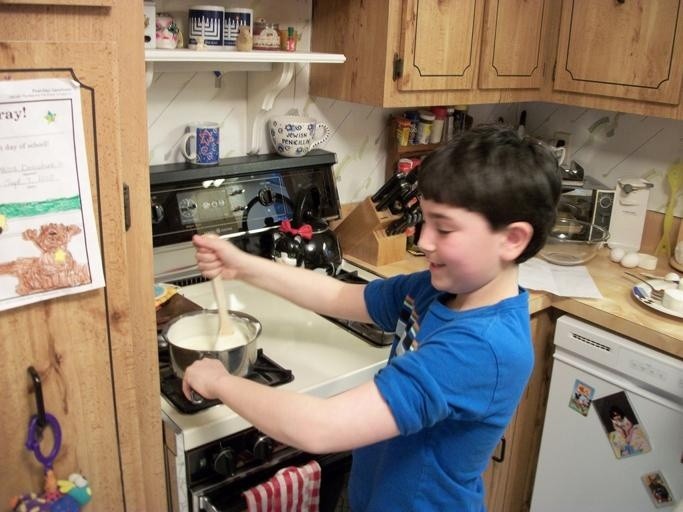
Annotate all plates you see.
[632,277,683,321]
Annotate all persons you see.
[648,476,669,502]
[180,127,561,512]
[579,387,591,409]
[609,405,633,443]
[575,385,584,408]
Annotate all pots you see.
[163,309,262,385]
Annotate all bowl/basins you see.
[542,218,611,264]
[662,288,683,314]
[637,253,658,270]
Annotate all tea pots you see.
[273,186,342,276]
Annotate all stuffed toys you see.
[9,467,92,512]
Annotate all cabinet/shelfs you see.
[1,0,683,510]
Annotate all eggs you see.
[610,249,638,267]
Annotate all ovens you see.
[184,427,352,512]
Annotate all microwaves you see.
[558,176,616,244]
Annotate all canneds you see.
[403,105,470,144]
[252,22,283,51]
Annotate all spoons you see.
[640,272,679,282]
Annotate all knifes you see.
[372,165,423,235]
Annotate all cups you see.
[269,114,330,158]
[188,5,254,52]
[181,121,221,166]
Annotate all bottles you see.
[285,27,296,51]
[408,105,468,145]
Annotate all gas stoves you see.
[157,270,413,415]
